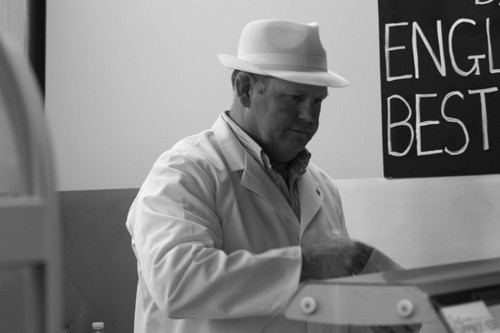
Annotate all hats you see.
[216,19,352,88]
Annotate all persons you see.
[125,20,425,333]
